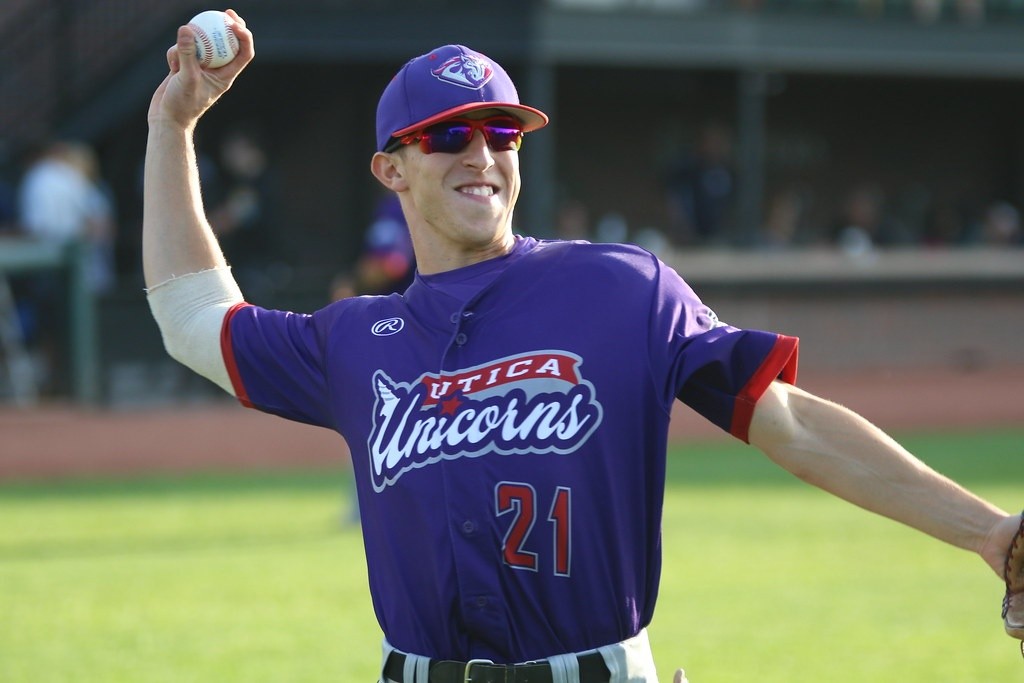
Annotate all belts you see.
[382,651,611,683]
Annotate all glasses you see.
[382,116,524,154]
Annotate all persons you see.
[556,100,1024,256]
[141,8,1024,683]
[17,123,417,409]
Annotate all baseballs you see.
[181,8,244,73]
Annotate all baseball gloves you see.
[1002,508,1024,638]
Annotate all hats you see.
[376,43,549,151]
